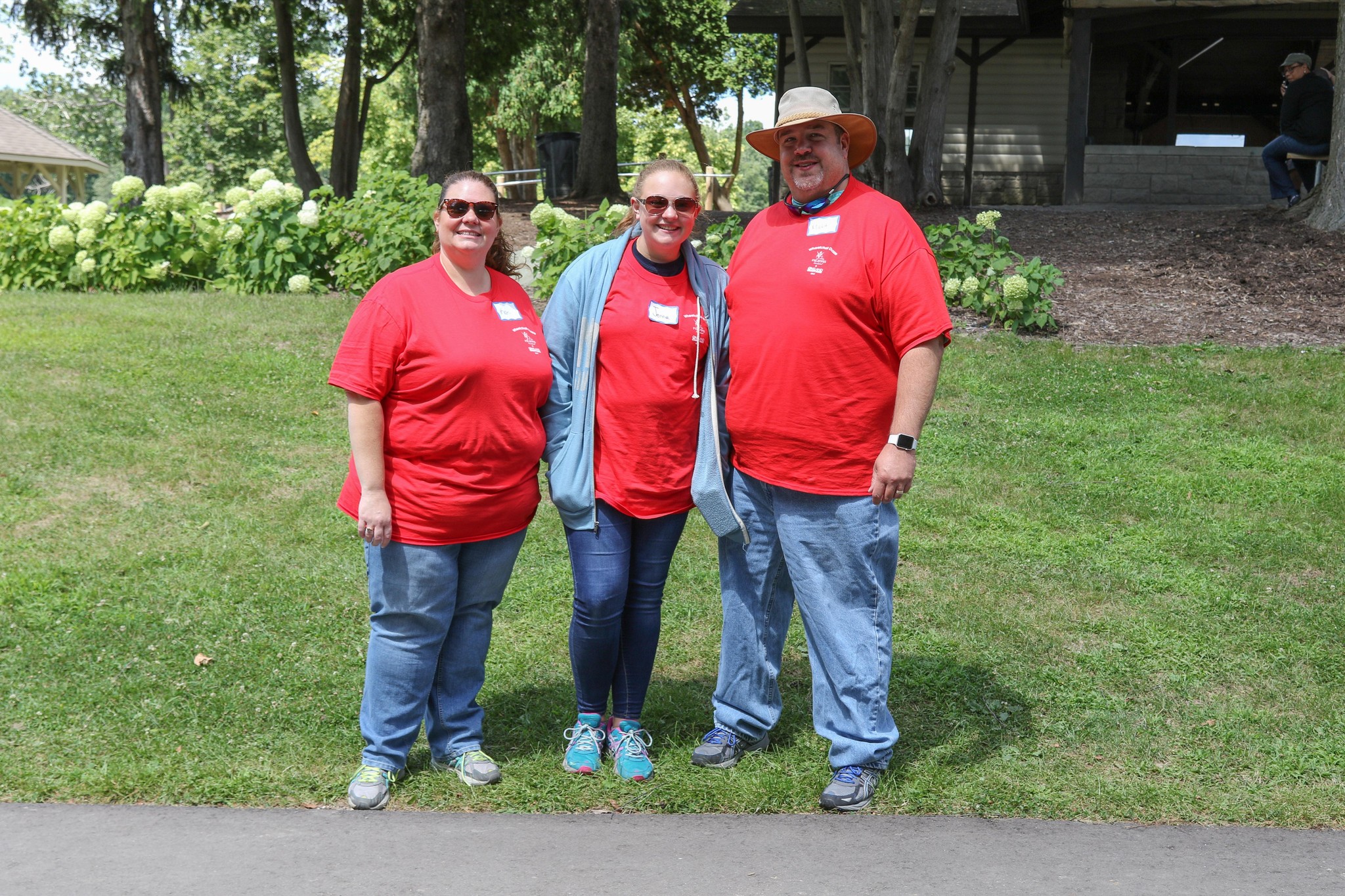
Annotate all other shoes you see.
[1287,192,1300,206]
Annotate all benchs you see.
[1285,150,1331,191]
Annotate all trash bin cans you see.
[535,131,580,199]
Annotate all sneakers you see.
[347,764,405,810]
[818,765,886,811]
[563,713,607,775]
[692,728,770,769]
[606,716,654,782]
[431,750,501,786]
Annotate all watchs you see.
[887,432,921,454]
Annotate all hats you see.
[1281,53,1312,69]
[745,86,877,168]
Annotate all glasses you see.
[438,198,499,221]
[636,195,698,217]
[784,174,850,217]
[1281,65,1299,77]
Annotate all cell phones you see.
[1284,79,1289,87]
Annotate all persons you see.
[539,159,732,789]
[330,169,554,813]
[690,83,945,807]
[1263,52,1336,208]
